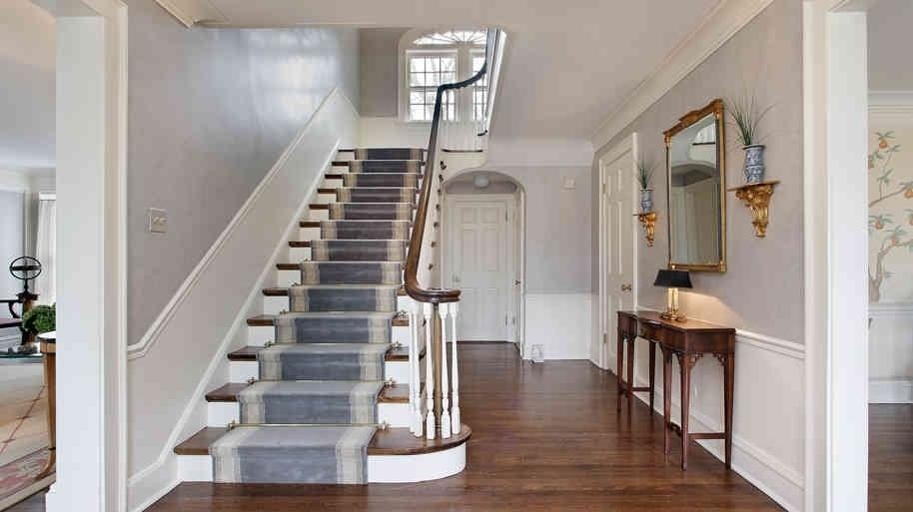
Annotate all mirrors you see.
[661,98,727,273]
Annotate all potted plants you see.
[715,90,777,184]
[630,151,661,212]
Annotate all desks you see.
[37,330,57,479]
[616,310,735,471]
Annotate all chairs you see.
[0,299,27,345]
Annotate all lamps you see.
[653,265,693,323]
[473,174,489,188]
